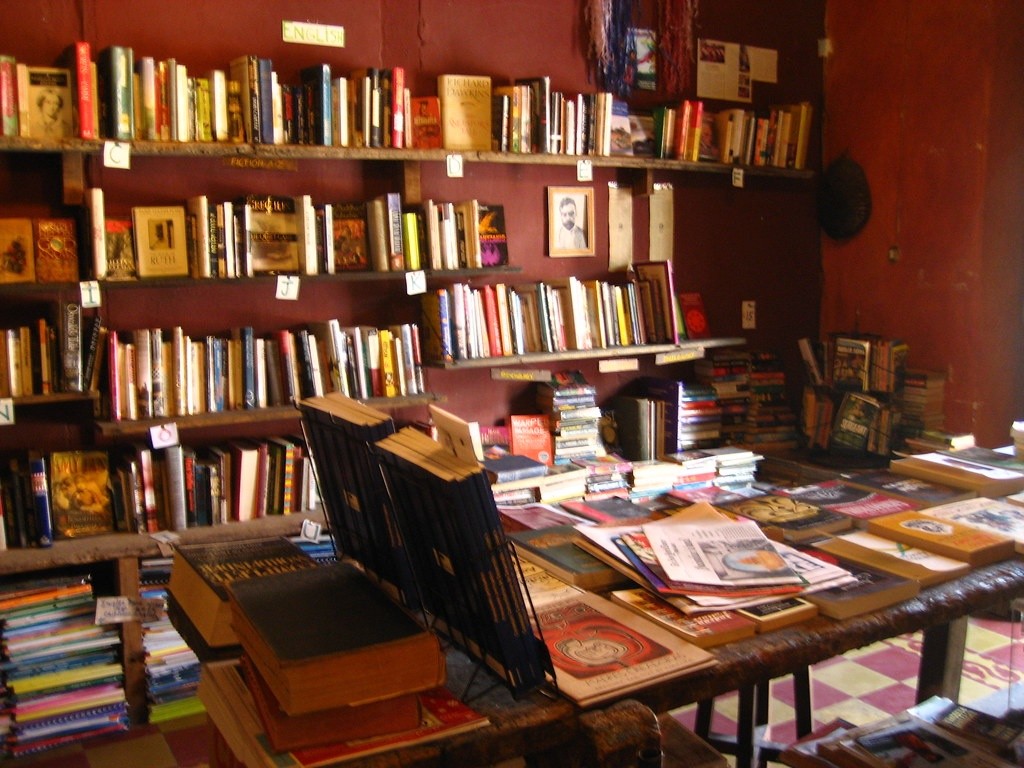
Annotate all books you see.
[0,302,425,425]
[0,187,508,283]
[0,391,547,768]
[421,259,711,359]
[0,40,813,170]
[416,332,1024,705]
[779,696,1024,768]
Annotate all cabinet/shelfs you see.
[1,139,815,767]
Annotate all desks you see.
[210,468,1024,768]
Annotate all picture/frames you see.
[546,185,595,258]
